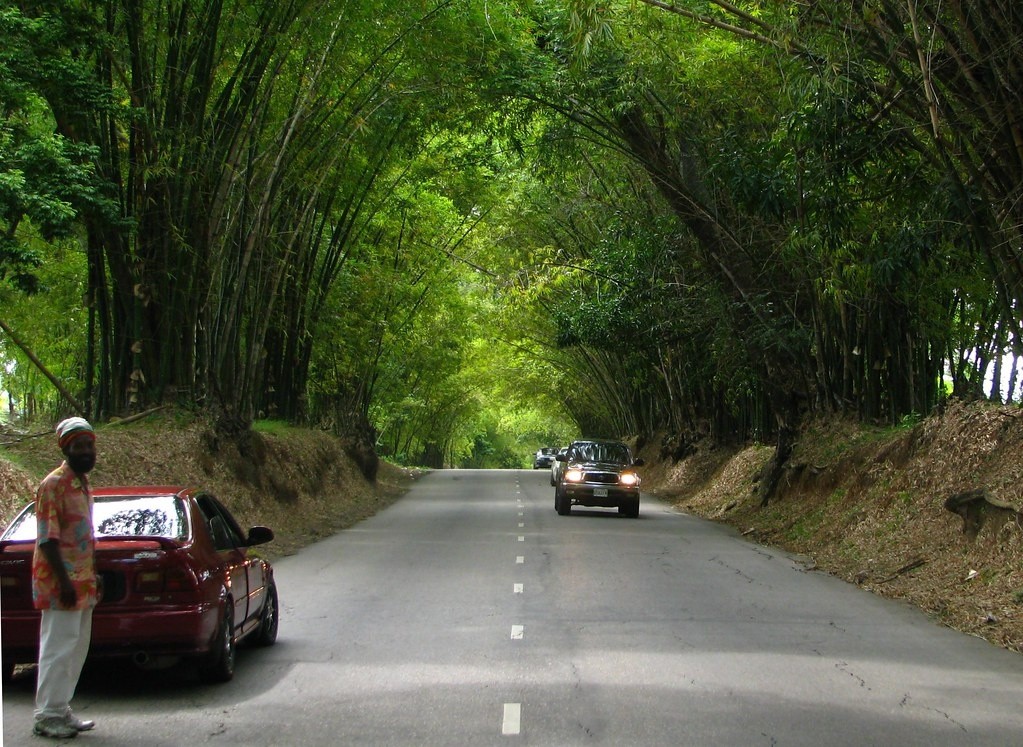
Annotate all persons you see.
[32,416,98,739]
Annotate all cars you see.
[0,484,278,690]
[549,446,569,487]
[532,447,560,469]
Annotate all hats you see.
[57,417,96,450]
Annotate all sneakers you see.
[33,716,78,739]
[63,709,95,732]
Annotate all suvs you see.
[555,438,645,517]
